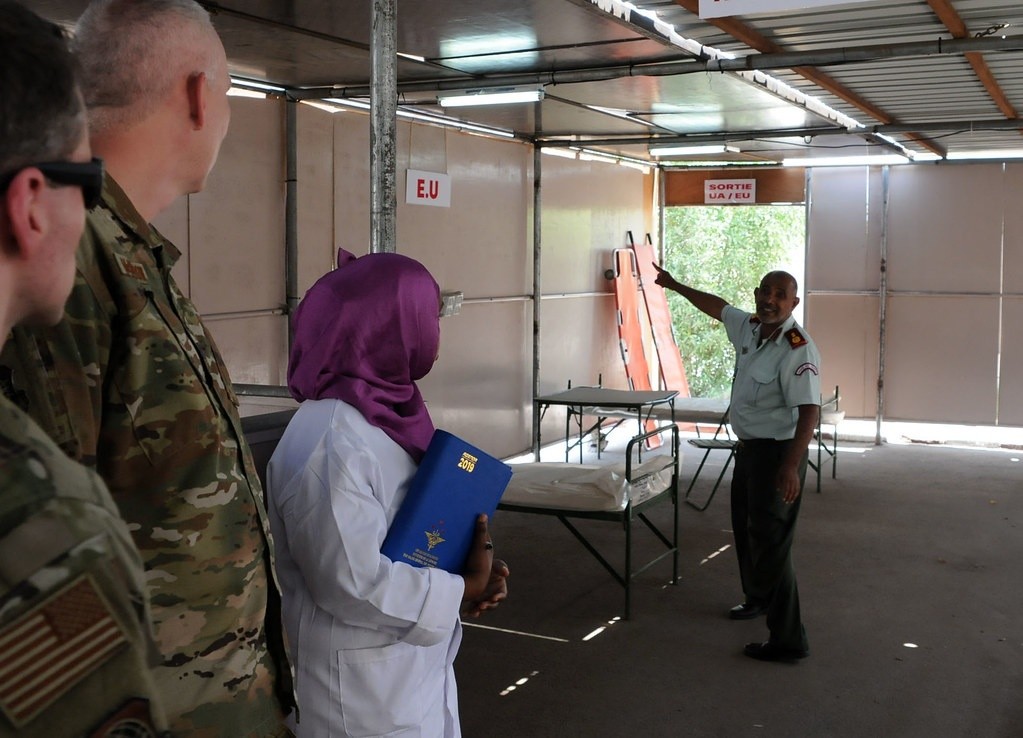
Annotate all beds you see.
[564,373,841,494]
[497,423,680,622]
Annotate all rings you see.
[486,542,493,551]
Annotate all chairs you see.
[684,401,744,512]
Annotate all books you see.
[376,428,510,574]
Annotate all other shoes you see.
[727,598,767,619]
[743,639,810,662]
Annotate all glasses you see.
[1,156,106,210]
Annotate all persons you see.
[1,2,306,738]
[651,262,820,662]
[1,6,180,736]
[262,251,511,738]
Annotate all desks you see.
[529,386,680,465]
